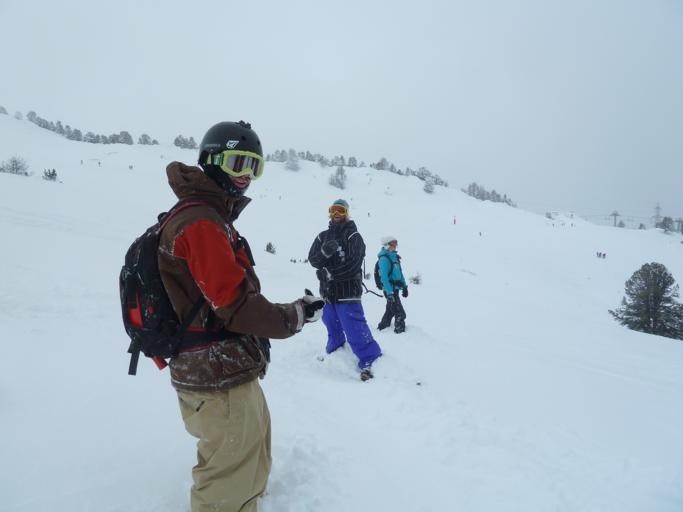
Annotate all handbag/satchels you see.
[119,199,210,358]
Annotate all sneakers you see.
[361,366,373,380]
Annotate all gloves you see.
[316,268,328,281]
[402,286,408,297]
[299,288,325,322]
[321,240,339,258]
[387,294,396,302]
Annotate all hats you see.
[382,236,397,245]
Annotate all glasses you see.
[328,205,346,216]
[206,149,264,179]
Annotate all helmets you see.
[199,120,264,165]
[333,198,349,209]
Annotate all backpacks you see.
[374,255,393,290]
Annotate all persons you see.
[376,239,408,334]
[308,198,382,382]
[151,121,326,512]
